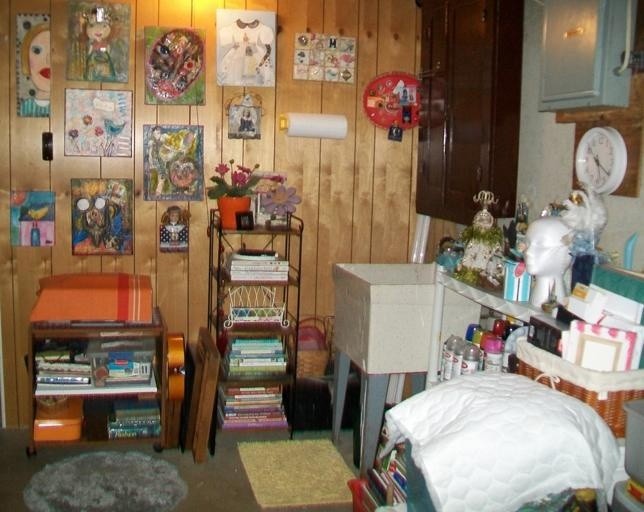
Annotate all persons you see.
[400,89,409,105]
[522,217,572,310]
[159,205,188,247]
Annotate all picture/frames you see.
[566,320,637,373]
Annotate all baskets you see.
[516,336,644,438]
[288,317,331,377]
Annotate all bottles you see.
[435,318,522,384]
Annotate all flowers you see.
[206,159,261,199]
[262,186,301,216]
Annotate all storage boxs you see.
[622,399,644,489]
[34,398,84,442]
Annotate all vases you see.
[219,196,251,230]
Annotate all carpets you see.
[239,440,357,509]
[23,451,187,512]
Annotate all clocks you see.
[575,127,628,196]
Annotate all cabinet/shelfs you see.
[423,263,550,390]
[26,307,169,454]
[415,0,524,227]
[209,208,304,440]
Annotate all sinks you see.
[331,263,483,375]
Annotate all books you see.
[33,338,162,441]
[372,425,408,506]
[215,246,290,431]
